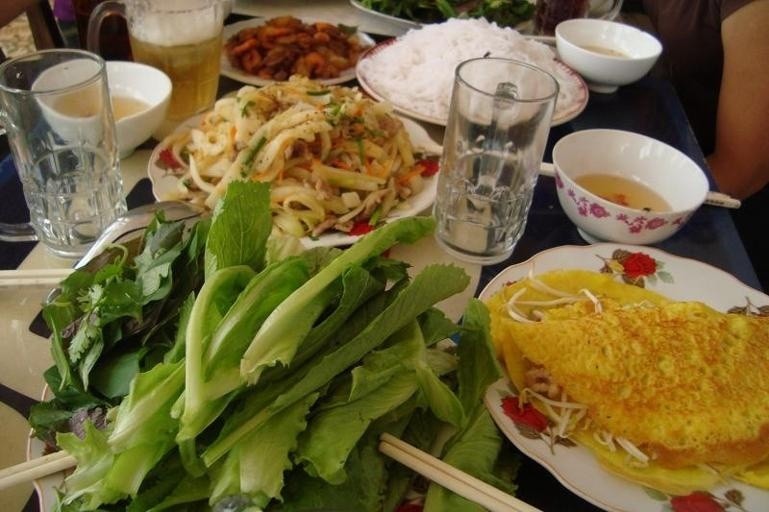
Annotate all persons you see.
[533,0,769,201]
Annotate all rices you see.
[356,17,578,123]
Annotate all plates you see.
[152,96,455,253]
[354,26,592,131]
[28,350,510,511]
[349,1,624,45]
[465,242,768,510]
[206,11,393,87]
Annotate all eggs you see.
[478,268,768,497]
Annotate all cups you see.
[83,1,228,151]
[2,48,140,258]
[430,56,564,268]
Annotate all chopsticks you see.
[376,428,543,510]
[531,156,745,210]
[0,449,81,488]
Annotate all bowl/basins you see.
[554,12,666,100]
[549,127,714,250]
[25,58,174,169]
